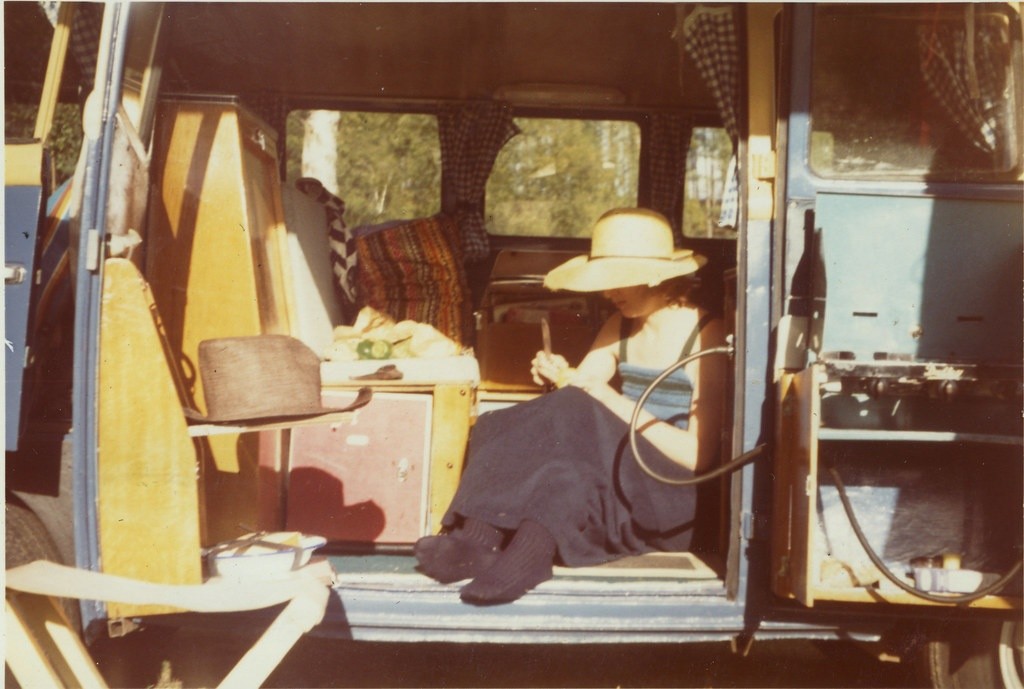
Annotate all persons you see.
[412,204,732,603]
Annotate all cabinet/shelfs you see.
[143,93,297,541]
[768,361,1024,614]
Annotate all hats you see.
[543,208,710,293]
[182,334,373,423]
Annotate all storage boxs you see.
[254,381,475,558]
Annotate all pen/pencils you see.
[541,319,553,361]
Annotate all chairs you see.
[475,244,597,391]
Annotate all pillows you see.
[353,214,475,351]
